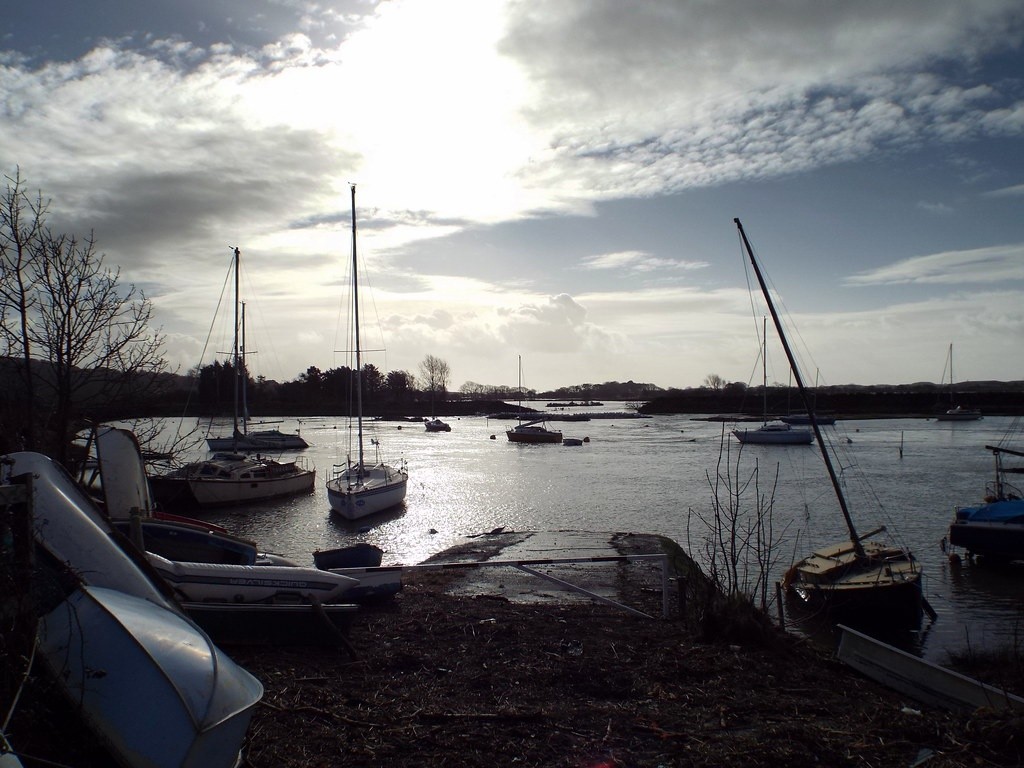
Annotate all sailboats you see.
[731,316,817,449]
[202,301,313,452]
[321,179,411,521]
[729,215,942,626]
[935,343,985,422]
[146,245,317,506]
[504,354,563,443]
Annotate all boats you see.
[308,540,386,569]
[424,419,452,433]
[945,443,1023,563]
[0,420,401,768]
[563,437,584,447]
